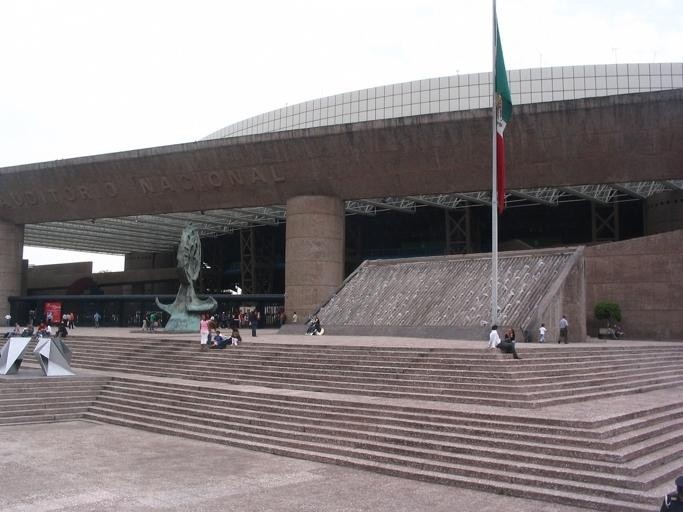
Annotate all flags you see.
[493,6,514,216]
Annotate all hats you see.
[675,476,683,492]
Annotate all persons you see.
[486,325,520,359]
[307,316,321,336]
[503,327,515,354]
[557,315,568,345]
[3,308,298,352]
[538,323,546,343]
